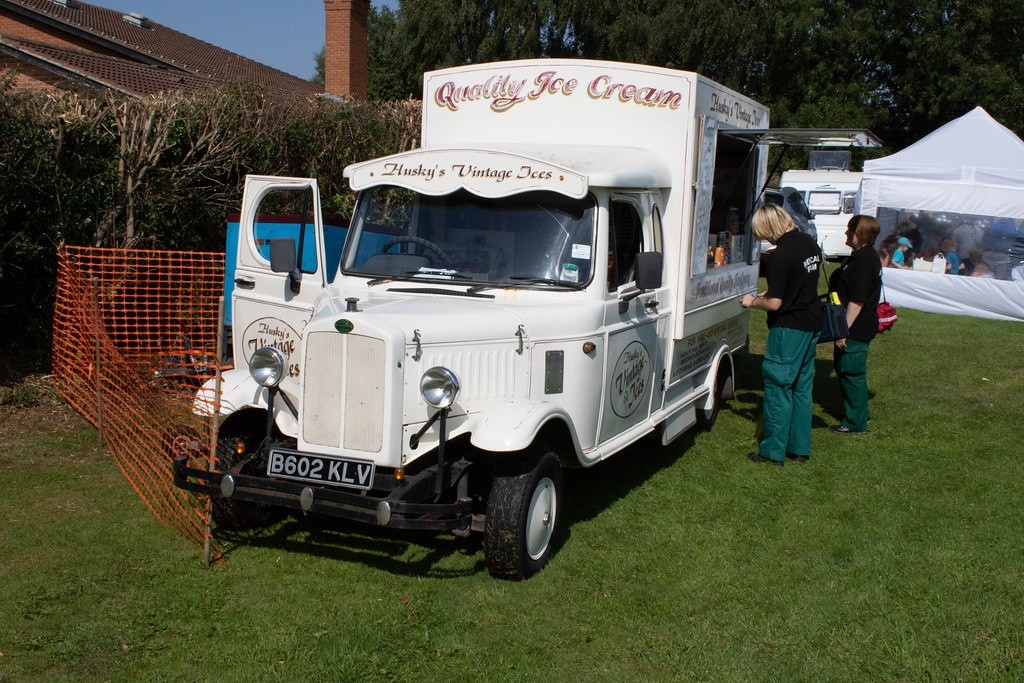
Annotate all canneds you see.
[714,247,724,266]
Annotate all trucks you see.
[172,59,885,582]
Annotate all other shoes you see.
[785,452,810,463]
[747,452,784,468]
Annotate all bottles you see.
[718,232,728,264]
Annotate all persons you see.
[740,204,822,466]
[940,237,966,275]
[827,215,882,434]
[971,218,1024,281]
[879,215,935,270]
[953,216,977,269]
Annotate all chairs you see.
[424,240,505,277]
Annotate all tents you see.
[854,104,1024,282]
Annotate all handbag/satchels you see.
[932,252,947,274]
[815,292,850,344]
[913,257,933,272]
[876,302,897,334]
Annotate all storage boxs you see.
[708,234,744,263]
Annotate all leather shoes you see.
[830,424,867,435]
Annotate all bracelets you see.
[751,298,757,306]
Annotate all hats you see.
[897,237,913,249]
